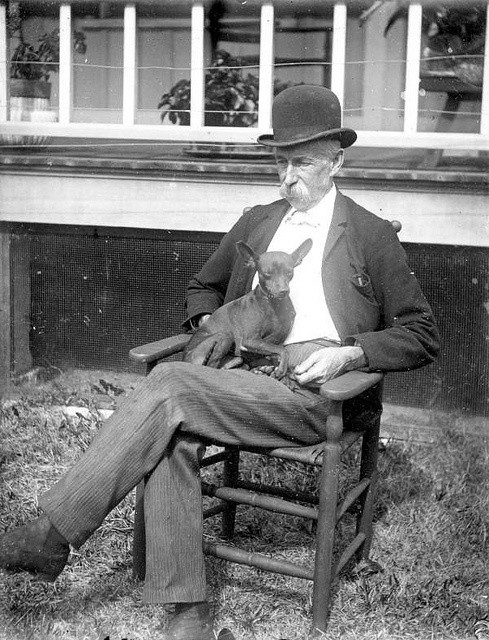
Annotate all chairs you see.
[125,204,405,636]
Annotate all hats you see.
[257,84,357,148]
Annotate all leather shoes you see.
[164,600,218,636]
[0,524,70,582]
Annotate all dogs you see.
[181,239,313,380]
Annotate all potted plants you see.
[0,1,88,120]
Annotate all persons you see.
[0,85,441,640]
